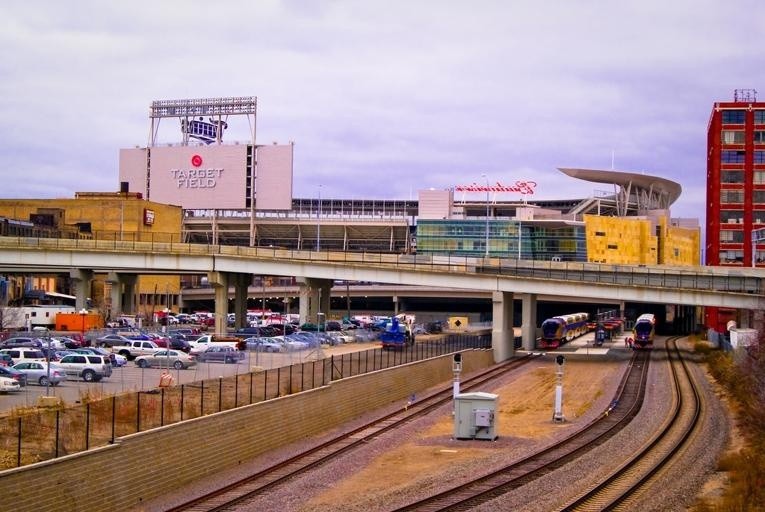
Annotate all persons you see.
[624,336,628,348]
[118,317,123,327]
[124,319,129,327]
[79,333,87,347]
[629,337,634,348]
[200,322,209,332]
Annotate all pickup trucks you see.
[190,334,240,354]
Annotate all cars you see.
[0,337,127,391]
[169,313,260,328]
[235,320,387,353]
[97,333,202,369]
[196,347,245,363]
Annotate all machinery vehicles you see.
[381,313,414,351]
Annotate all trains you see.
[541,313,590,348]
[632,314,655,350]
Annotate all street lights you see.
[79,308,89,337]
[482,173,491,257]
